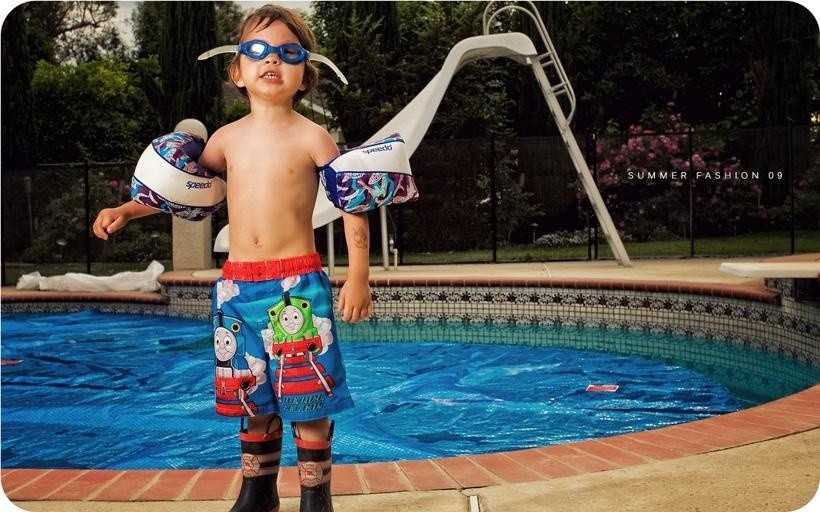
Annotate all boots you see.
[228,415,283,512]
[291,420,335,512]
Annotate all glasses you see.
[198,40,348,85]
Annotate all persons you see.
[92,3,375,511]
